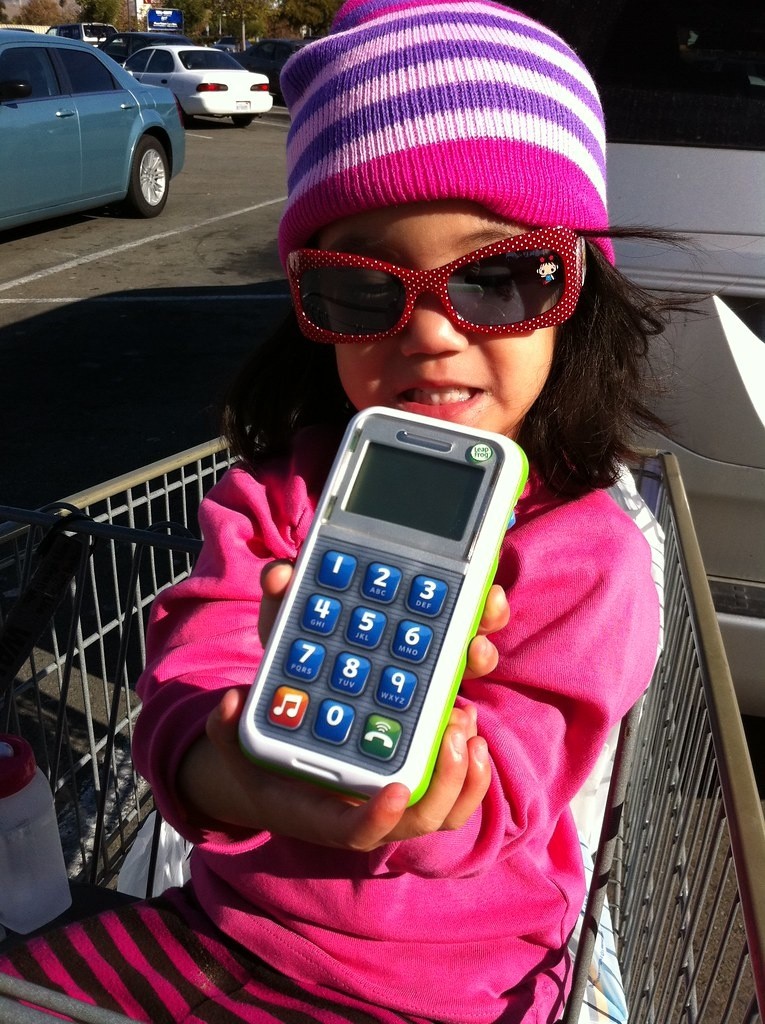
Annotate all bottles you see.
[0,731,73,933]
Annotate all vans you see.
[44,22,123,51]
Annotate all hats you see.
[277,0,615,269]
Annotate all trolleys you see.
[0,426,765,1024]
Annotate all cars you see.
[0,29,186,230]
[117,45,274,127]
[99,33,195,70]
[229,38,317,103]
[215,36,253,53]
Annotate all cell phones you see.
[237,406,529,809]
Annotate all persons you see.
[0,0,726,1024]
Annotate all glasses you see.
[287,225,587,345]
